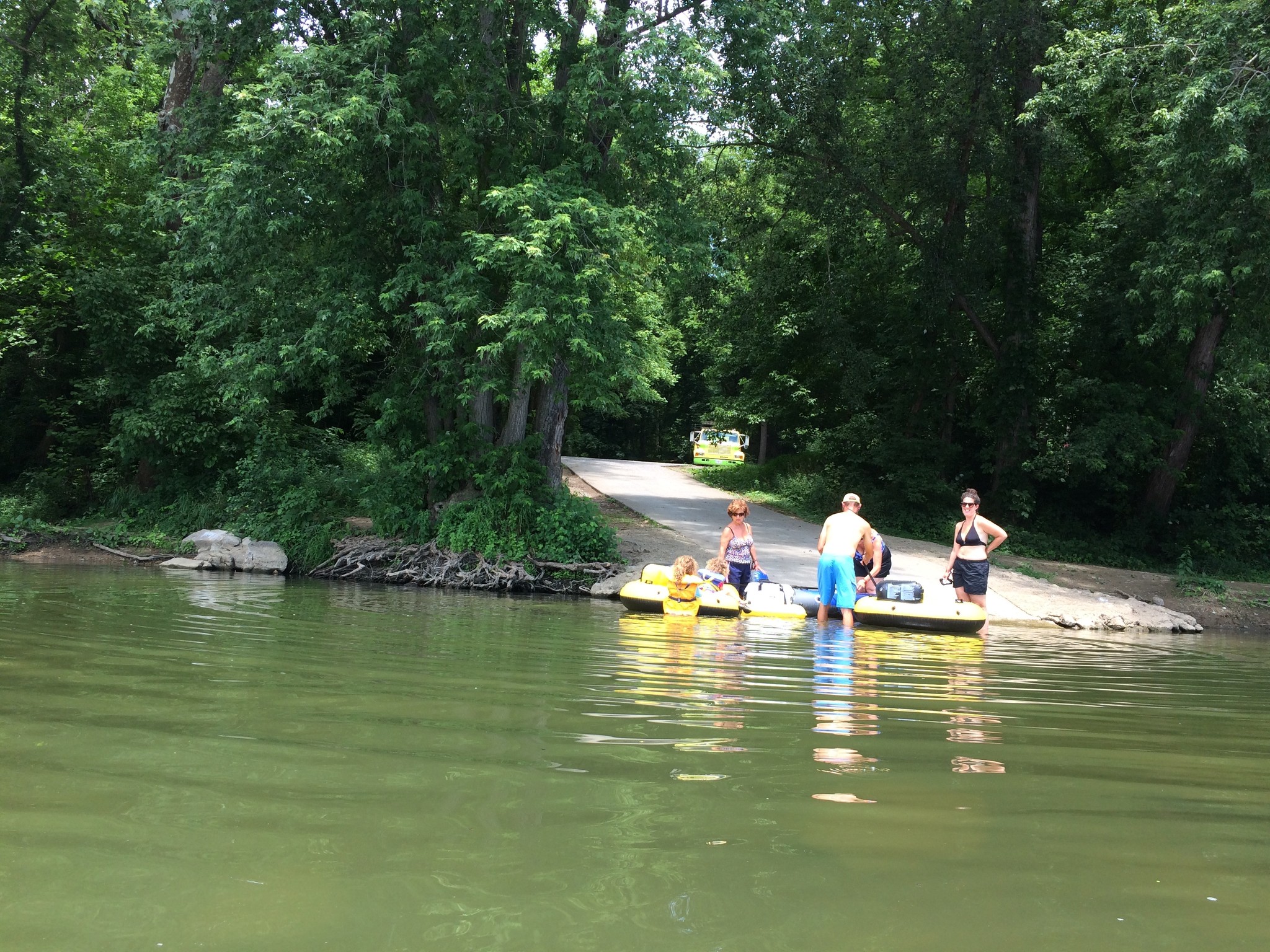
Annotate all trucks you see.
[690,419,749,468]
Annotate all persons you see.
[942,488,1007,629]
[818,493,874,626]
[663,555,751,617]
[718,499,767,601]
[853,528,892,594]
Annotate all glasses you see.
[731,511,745,516]
[861,538,863,540]
[961,502,975,508]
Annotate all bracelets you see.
[946,569,951,574]
[862,578,867,584]
[751,559,759,570]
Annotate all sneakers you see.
[738,603,752,614]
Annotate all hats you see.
[842,493,860,511]
